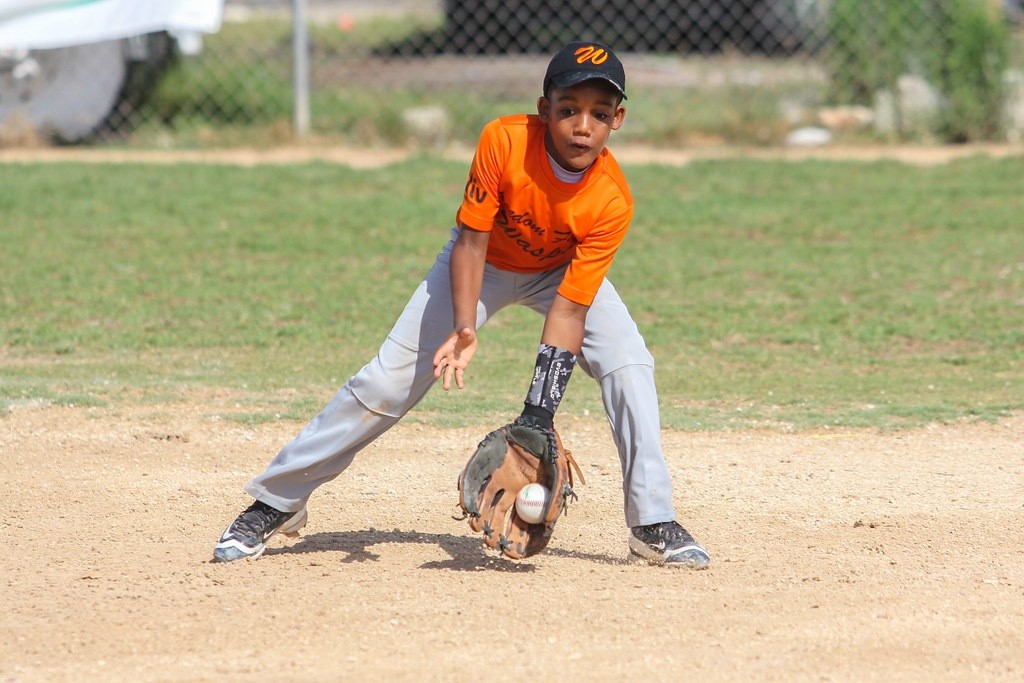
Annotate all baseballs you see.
[513,482,553,524]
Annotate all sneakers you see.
[627,521,710,570]
[214,500,308,562]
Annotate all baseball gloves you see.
[455,401,573,560]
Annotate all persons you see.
[213,42,711,571]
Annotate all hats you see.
[543,41,628,100]
